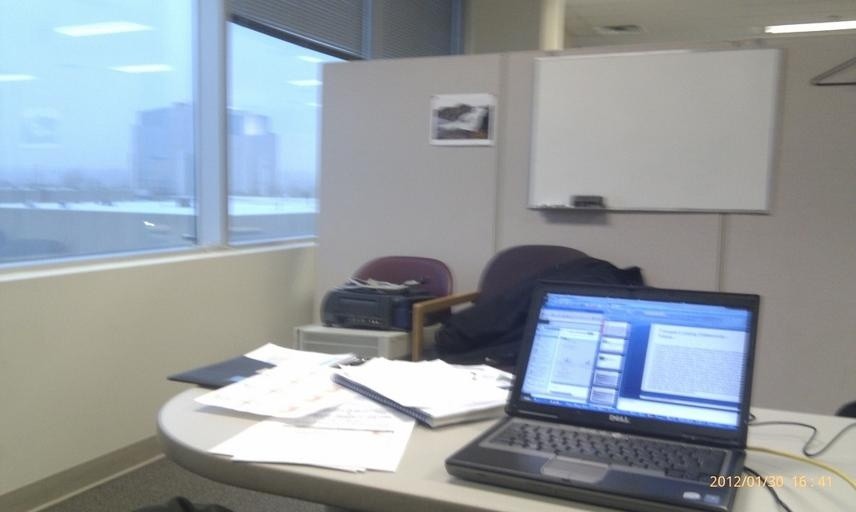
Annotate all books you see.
[166,355,279,389]
[334,357,505,428]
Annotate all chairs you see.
[411,244,616,364]
[351,255,454,320]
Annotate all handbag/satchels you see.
[432,256,645,365]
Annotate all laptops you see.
[443,276,761,511]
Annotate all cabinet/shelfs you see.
[292,321,443,360]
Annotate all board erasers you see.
[571,195,603,207]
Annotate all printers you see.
[319,284,441,333]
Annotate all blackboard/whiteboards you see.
[528,48,780,216]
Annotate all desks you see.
[156,356,856,512]
